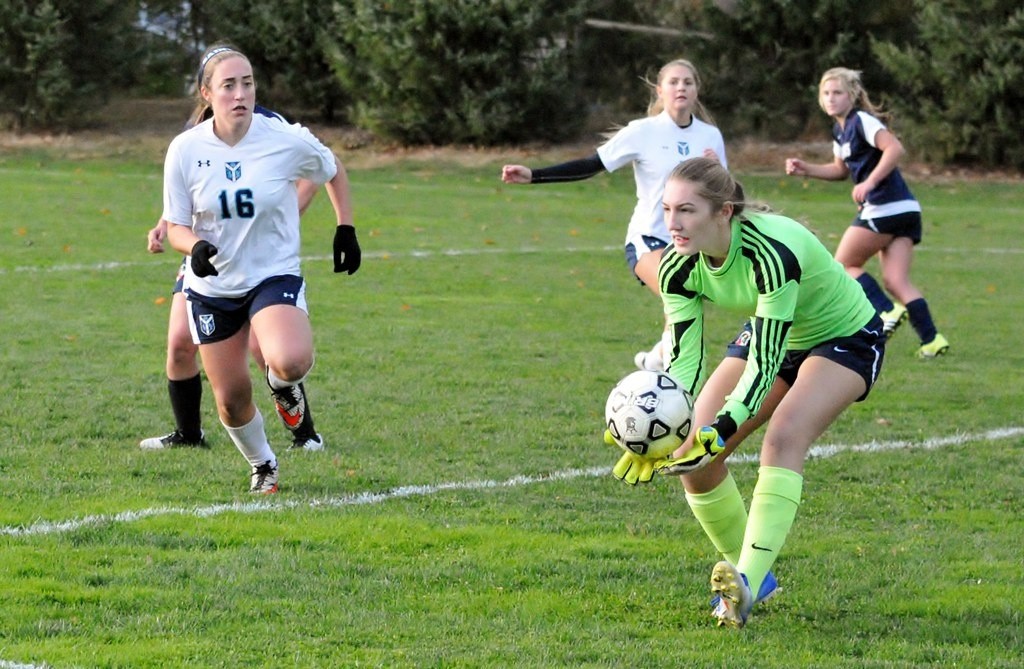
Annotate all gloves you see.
[603,429,671,485]
[333,224,361,275]
[658,414,737,476]
[190,240,218,278]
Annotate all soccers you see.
[605,370,694,459]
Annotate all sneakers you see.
[248,453,279,495]
[710,561,753,630]
[754,571,783,606]
[286,434,324,455]
[266,364,305,430]
[879,303,908,343]
[914,333,949,361]
[140,428,205,452]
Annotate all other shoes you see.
[635,352,661,372]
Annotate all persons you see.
[605,158,886,630]
[503,58,731,377]
[140,44,326,458]
[163,50,362,495]
[785,68,951,360]
